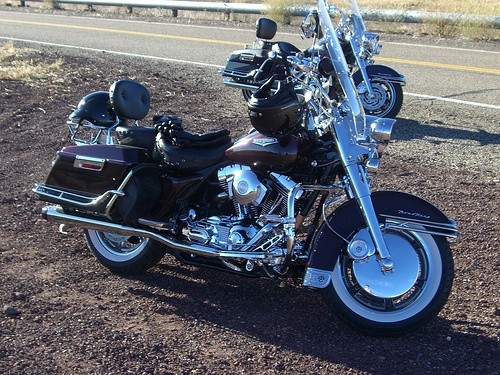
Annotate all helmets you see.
[248,74,302,138]
[68,91,128,128]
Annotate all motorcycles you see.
[219,0,406,119]
[31,0,458,335]
